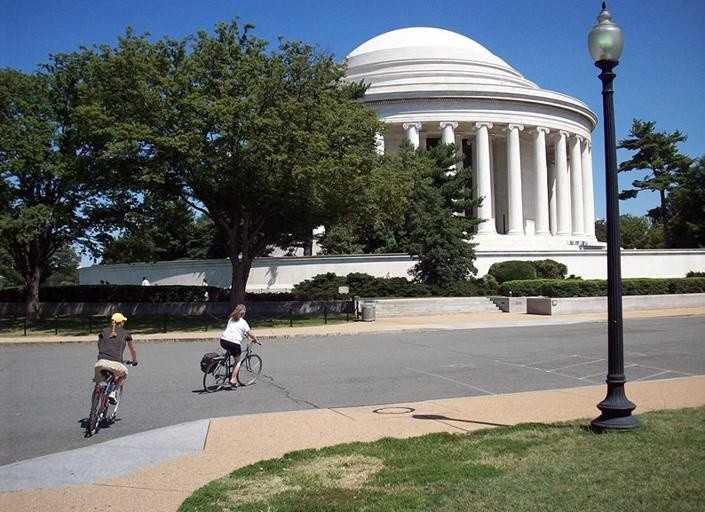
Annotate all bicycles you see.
[86,359,137,437]
[201,337,264,393]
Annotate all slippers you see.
[228,381,238,387]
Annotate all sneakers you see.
[108,393,117,405]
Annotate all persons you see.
[141,277,150,287]
[202,279,209,287]
[90,312,137,419]
[220,304,258,388]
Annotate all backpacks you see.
[200,353,220,373]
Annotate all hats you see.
[111,313,127,322]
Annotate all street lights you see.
[584,0,643,432]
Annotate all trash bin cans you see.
[362,303,375,322]
